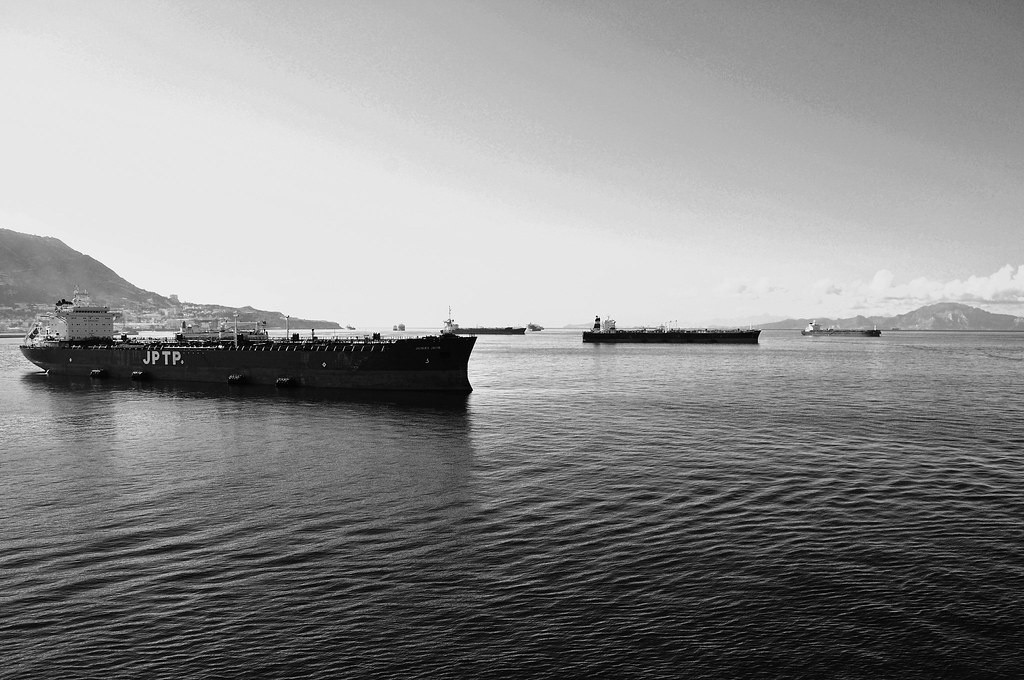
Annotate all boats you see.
[16,303,479,378]
[582,324,762,344]
[442,305,525,336]
[801,320,883,338]
